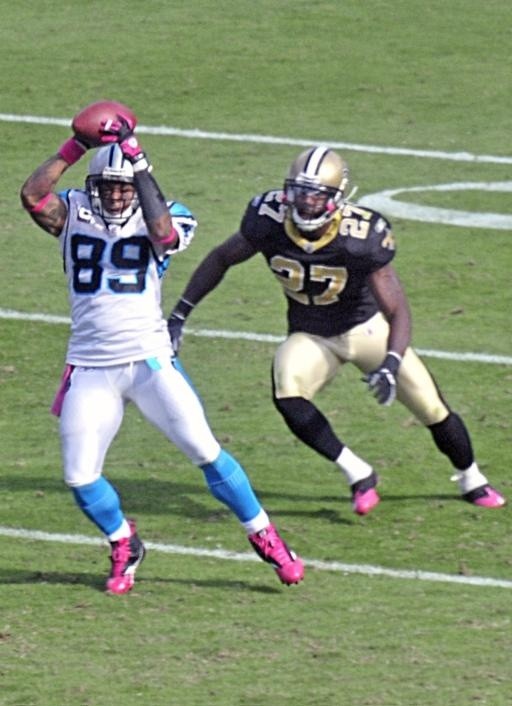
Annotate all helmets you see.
[84,142,155,226]
[283,145,359,240]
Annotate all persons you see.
[18,102,305,595]
[167,144,508,517]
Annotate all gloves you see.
[57,134,91,165]
[167,297,195,353]
[96,111,149,174]
[360,351,403,407]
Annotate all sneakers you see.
[104,518,147,595]
[246,523,306,588]
[349,467,379,515]
[460,483,505,509]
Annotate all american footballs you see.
[72,101,137,143]
